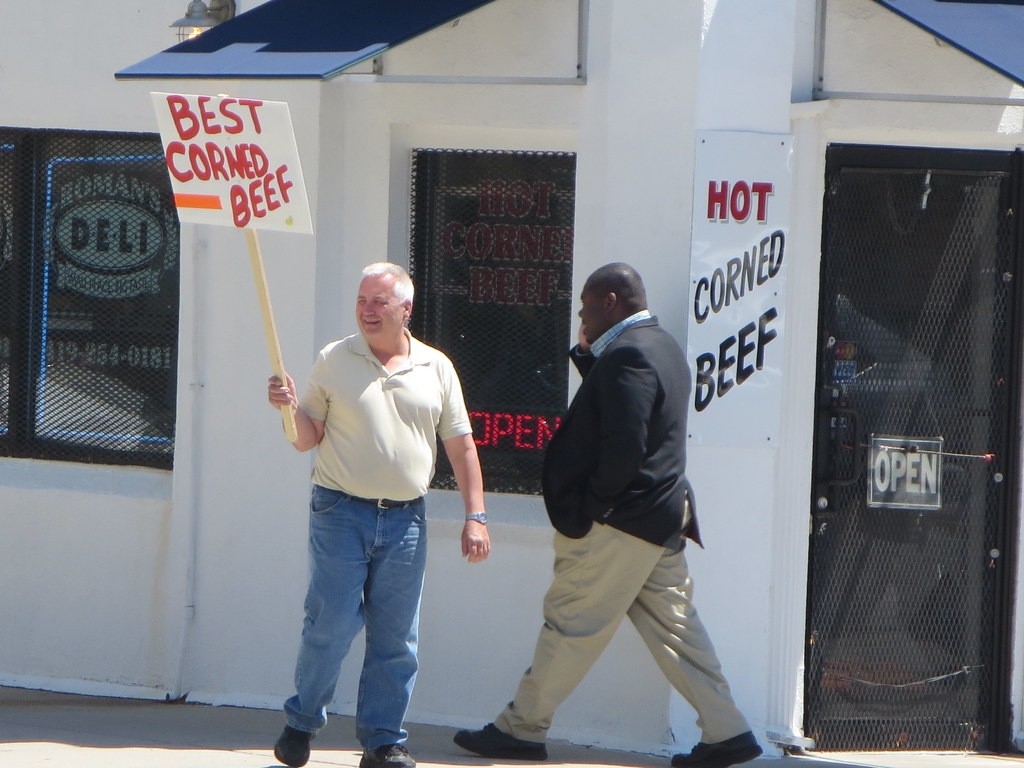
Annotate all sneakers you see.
[671,730,764,768]
[274,724,317,767]
[359,744,416,768]
[453,722,549,760]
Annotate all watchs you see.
[464,512,487,524]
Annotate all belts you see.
[338,490,424,509]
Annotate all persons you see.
[268,262,492,768]
[451,262,762,768]
